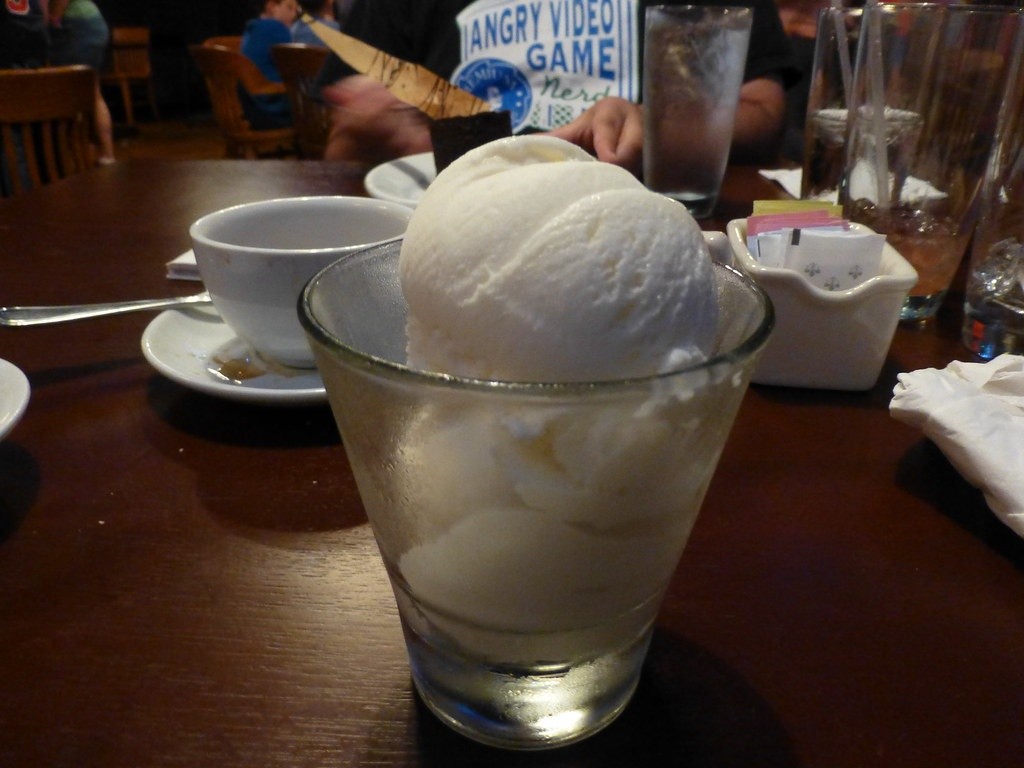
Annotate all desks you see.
[0,158,1024,768]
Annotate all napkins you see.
[759,159,946,210]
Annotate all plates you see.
[140,292,326,402]
[363,151,436,209]
[0,357,31,442]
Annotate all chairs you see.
[0,28,332,192]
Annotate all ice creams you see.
[397,133,728,634]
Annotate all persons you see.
[0,5,847,232]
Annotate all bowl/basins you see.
[188,196,413,368]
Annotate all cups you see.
[839,0,1020,327]
[800,5,863,206]
[643,4,752,219]
[296,238,777,749]
[727,218,918,390]
[961,5,1024,360]
